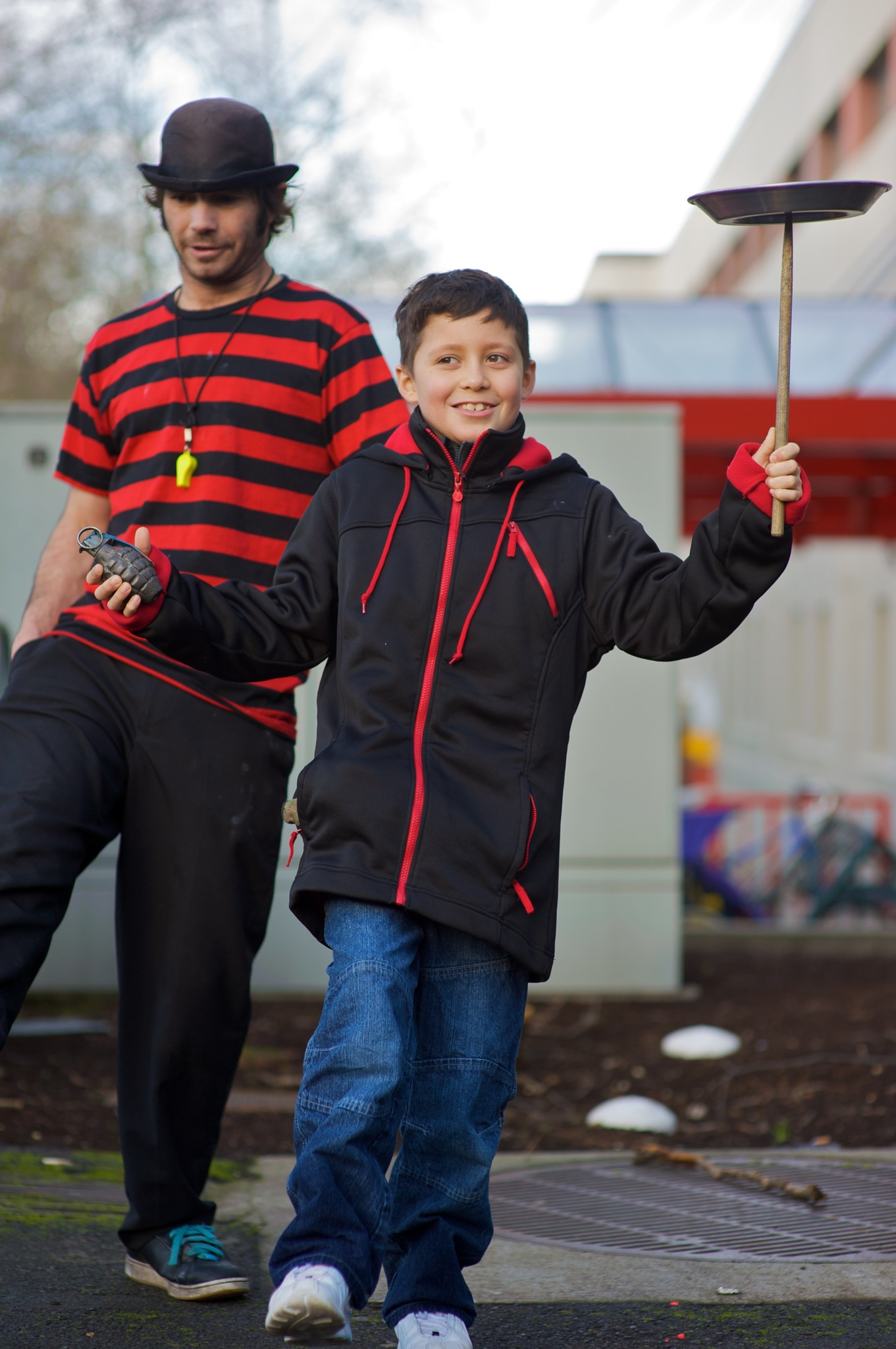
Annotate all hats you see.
[137,97,299,192]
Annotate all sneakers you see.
[394,1309,474,1349]
[125,1224,250,1300]
[265,1263,353,1344]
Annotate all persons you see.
[0,97,409,1303]
[87,269,809,1349]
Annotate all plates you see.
[687,181,893,227]
